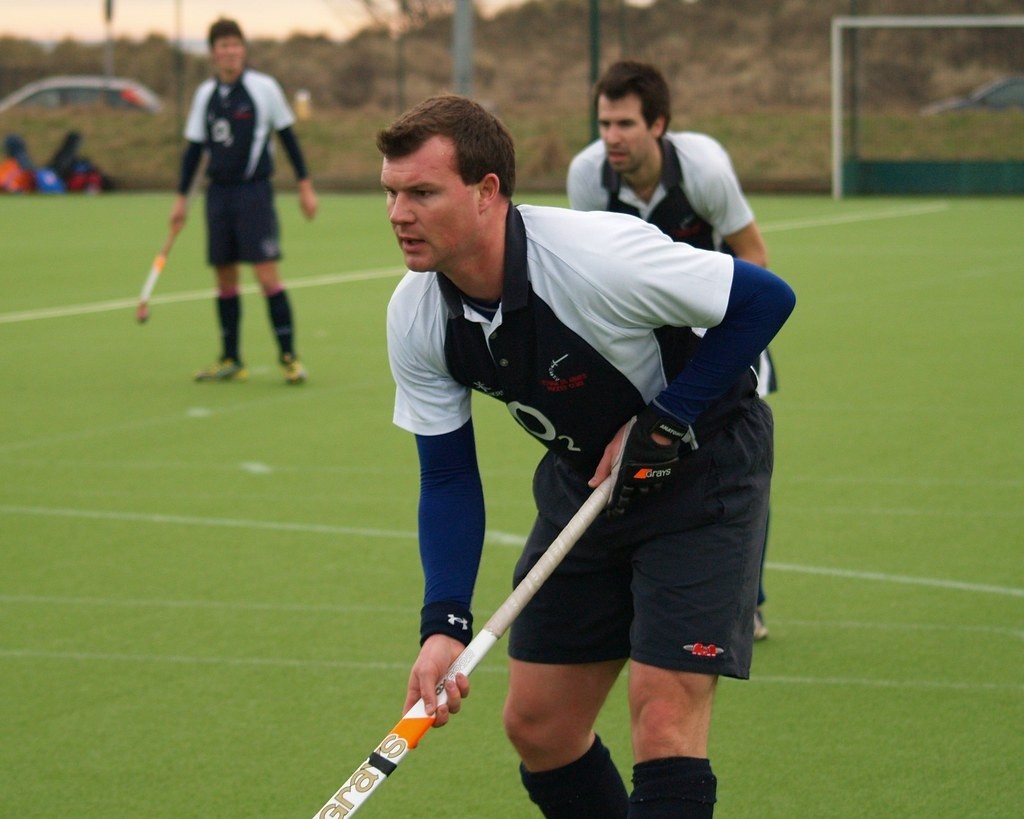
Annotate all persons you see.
[566,61,768,272]
[375,96,796,819]
[169,17,317,386]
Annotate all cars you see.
[917,76,1023,122]
[1,74,167,114]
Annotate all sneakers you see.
[282,355,305,383]
[193,359,248,382]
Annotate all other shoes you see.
[753,610,767,640]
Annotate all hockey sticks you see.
[308,473,612,819]
[134,225,178,323]
